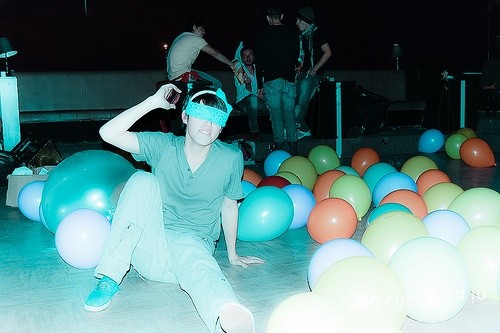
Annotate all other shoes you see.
[250,131,261,141]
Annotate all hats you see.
[296,6,314,25]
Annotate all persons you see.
[84,82,266,333]
[233,46,271,140]
[254,5,297,156]
[283,7,333,142]
[167,20,236,92]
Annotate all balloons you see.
[17,149,144,270]
[220,127,500,333]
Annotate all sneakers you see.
[219,302,254,333]
[84,278,120,312]
[297,128,311,139]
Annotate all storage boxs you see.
[5,166,56,207]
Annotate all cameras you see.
[166,89,180,104]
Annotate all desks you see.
[304,80,360,140]
